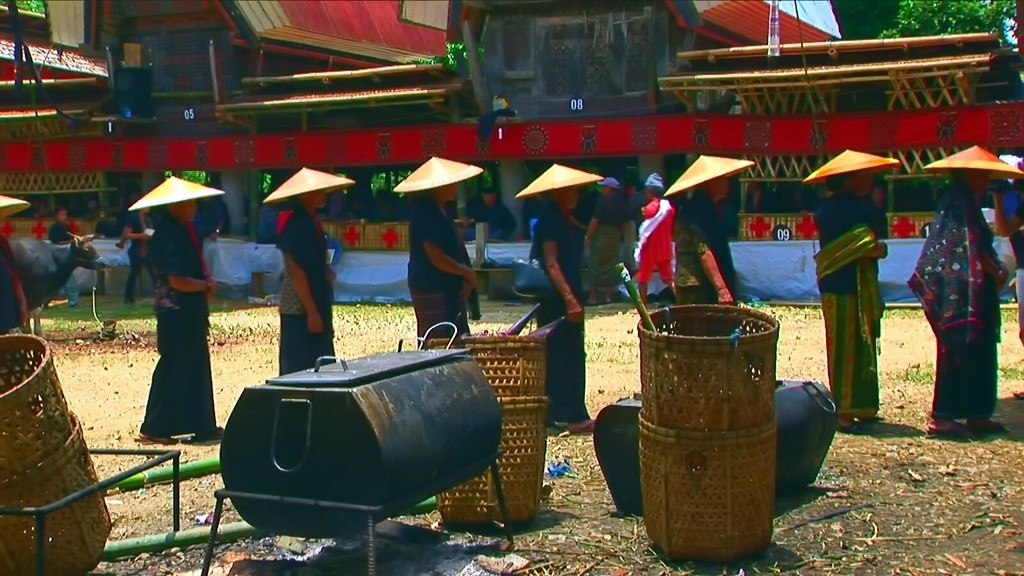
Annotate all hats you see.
[0,142,1023,221]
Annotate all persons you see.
[37,205,80,310]
[0,205,32,337]
[122,204,151,305]
[583,177,626,306]
[275,176,338,377]
[992,159,1024,398]
[465,187,518,243]
[139,186,226,445]
[628,173,680,315]
[907,150,1010,435]
[408,163,477,351]
[674,159,737,306]
[812,155,888,431]
[528,172,597,437]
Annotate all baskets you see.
[423,334,549,528]
[637,302,780,562]
[0,331,115,576]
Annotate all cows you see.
[6,231,110,340]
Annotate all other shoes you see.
[856,415,887,424]
[838,418,865,435]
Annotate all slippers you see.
[964,416,1003,432]
[926,418,972,436]
